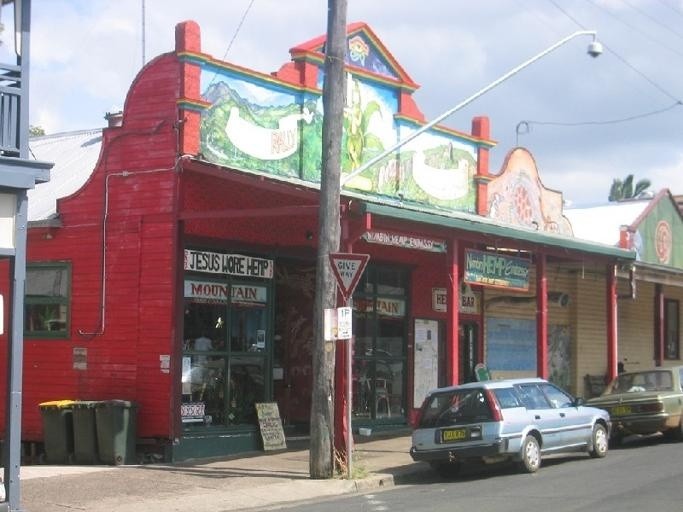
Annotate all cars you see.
[587,365,682,445]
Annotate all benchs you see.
[584,372,612,397]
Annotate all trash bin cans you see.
[39,400,71,463]
[71,400,96,464]
[94,400,143,466]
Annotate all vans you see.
[409,376,611,475]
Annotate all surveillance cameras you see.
[587,42,603,58]
